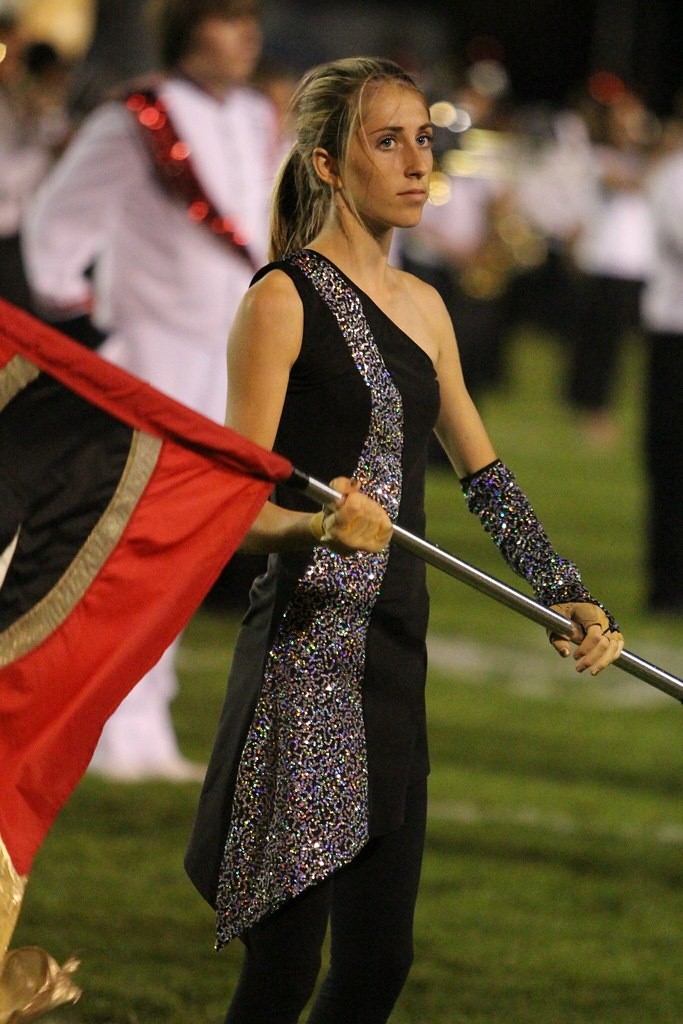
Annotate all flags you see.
[0,304,294,1024]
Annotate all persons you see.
[0,1,683,786]
[181,58,623,1024]
